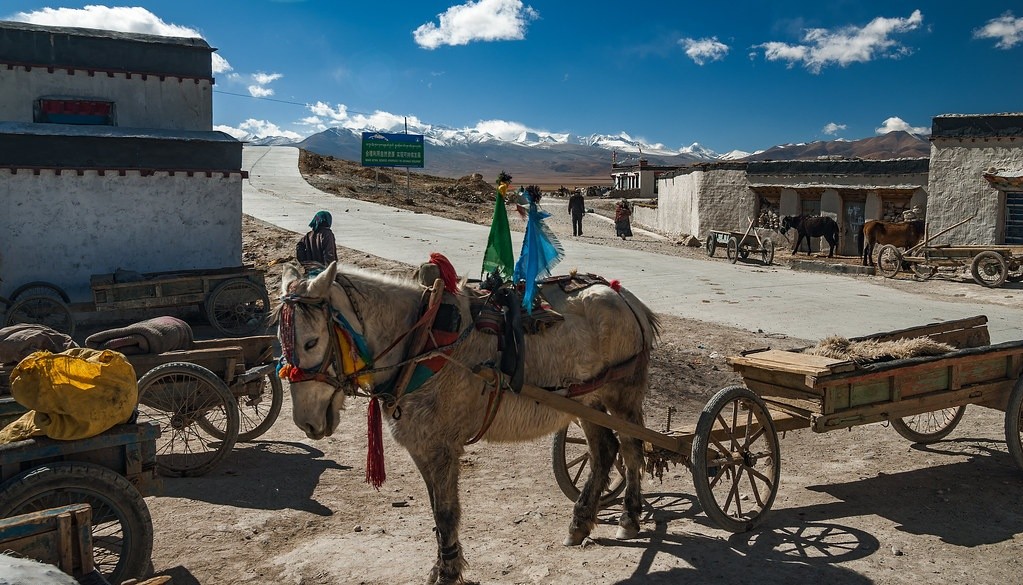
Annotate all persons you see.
[295,210,338,276]
[615,199,633,240]
[568,189,586,236]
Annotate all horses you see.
[857,220,925,271]
[266,259,664,585]
[780,213,839,258]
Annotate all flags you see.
[481,182,515,277]
[513,190,564,316]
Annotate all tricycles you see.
[0,500,173,585]
[0,335,285,477]
[0,395,162,585]
[706,216,774,263]
[879,210,1023,289]
[0,263,271,341]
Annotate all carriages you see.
[272,180,1023,584]
